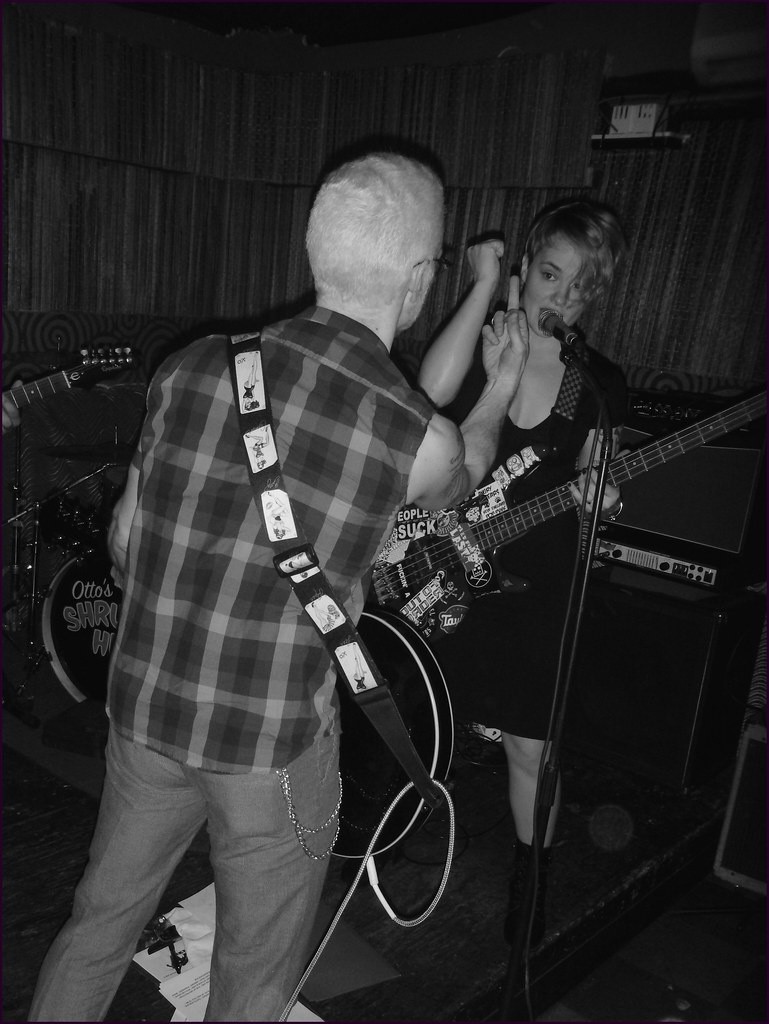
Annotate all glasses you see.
[413,258,451,278]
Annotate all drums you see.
[44,545,126,701]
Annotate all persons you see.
[419,197,628,878]
[27,151,529,1022]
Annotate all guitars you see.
[372,391,768,637]
[0,338,133,406]
[332,609,453,856]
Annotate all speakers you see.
[602,386,769,567]
[563,556,761,799]
[712,724,767,894]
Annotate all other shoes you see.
[504,836,552,957]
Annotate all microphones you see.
[537,308,589,365]
